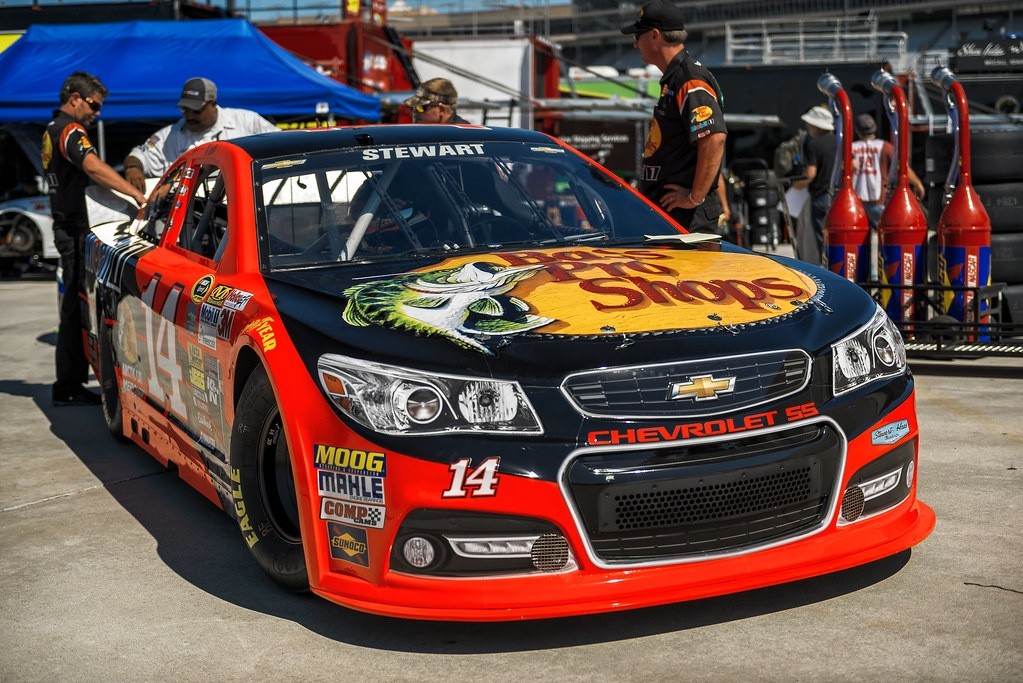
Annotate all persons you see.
[621,0,735,242]
[351,174,438,253]
[40,70,149,405]
[123,77,283,194]
[404,77,469,125]
[849,114,926,282]
[793,106,843,265]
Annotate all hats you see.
[403,77,457,108]
[620,0,684,35]
[856,115,877,136]
[800,106,835,131]
[176,78,218,111]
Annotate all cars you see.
[0,196,62,259]
[78,124,936,622]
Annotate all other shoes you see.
[52,382,101,406]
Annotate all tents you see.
[0,16,381,165]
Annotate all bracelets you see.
[126,163,142,169]
[689,193,705,206]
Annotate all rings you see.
[670,197,672,200]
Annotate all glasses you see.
[631,32,648,42]
[179,107,209,114]
[415,103,441,114]
[70,89,102,113]
